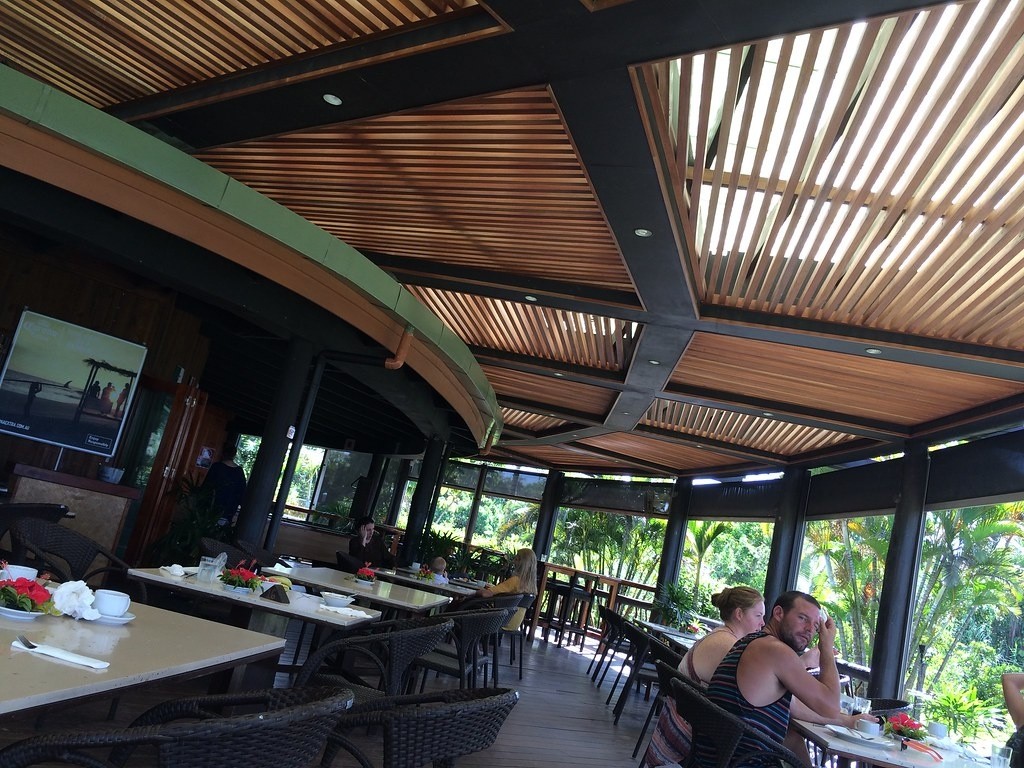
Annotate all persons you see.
[693,590,879,768]
[425,557,449,584]
[199,445,247,528]
[646,586,838,768]
[999,674,1024,768]
[348,518,408,569]
[90,381,129,417]
[24,382,42,416]
[477,548,538,631]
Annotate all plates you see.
[97,612,137,624]
[409,566,420,571]
[0,606,45,620]
[223,584,251,593]
[356,579,375,585]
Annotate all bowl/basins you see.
[257,579,280,593]
[320,592,356,607]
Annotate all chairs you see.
[808,672,850,689]
[0,591,537,768]
[0,501,367,604]
[586,604,810,768]
[865,697,913,719]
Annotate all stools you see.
[544,571,601,651]
[481,549,507,585]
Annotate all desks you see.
[526,561,622,654]
[632,617,991,768]
[0,569,493,718]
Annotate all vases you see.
[0,606,50,619]
[221,582,251,593]
[421,578,432,582]
[355,579,375,585]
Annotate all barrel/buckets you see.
[97,463,127,483]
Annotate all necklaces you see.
[725,625,735,634]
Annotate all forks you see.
[17,635,37,649]
[842,723,874,741]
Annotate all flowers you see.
[216,558,265,593]
[0,576,60,617]
[343,561,377,582]
[413,564,435,583]
[686,623,699,634]
[887,711,929,740]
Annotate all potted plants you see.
[141,472,235,602]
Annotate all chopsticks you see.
[825,723,896,749]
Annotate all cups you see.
[852,695,872,716]
[928,721,948,739]
[0,564,38,582]
[197,556,219,583]
[413,562,420,569]
[93,590,131,617]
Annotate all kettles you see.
[856,718,879,737]
